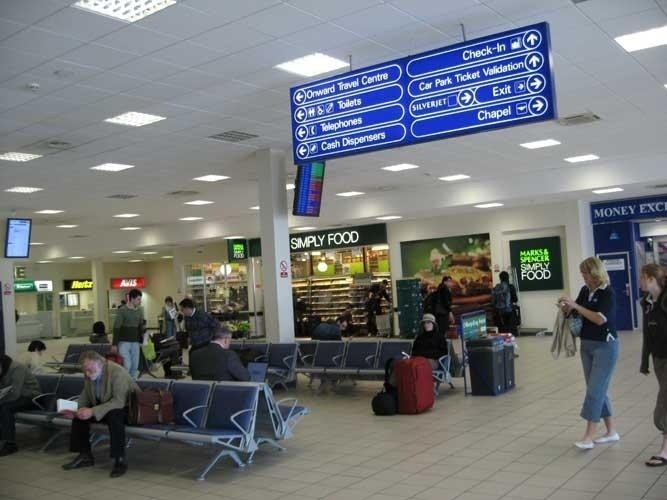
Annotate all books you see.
[56,399,78,417]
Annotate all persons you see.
[292,279,392,341]
[180,298,220,349]
[437,276,455,339]
[89,321,110,343]
[495,271,518,337]
[1,354,40,456]
[14,341,46,368]
[189,327,244,381]
[112,289,144,377]
[639,264,667,467]
[384,313,448,392]
[165,296,178,337]
[61,351,140,477]
[558,256,620,450]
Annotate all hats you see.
[422,314,435,323]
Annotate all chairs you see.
[260,383,310,451]
[14,376,260,481]
[44,344,112,373]
[229,340,243,351]
[448,340,464,389]
[243,340,268,363]
[261,340,447,396]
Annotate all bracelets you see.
[575,303,578,309]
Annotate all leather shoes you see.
[62,454,96,469]
[110,456,128,477]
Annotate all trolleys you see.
[373,310,393,338]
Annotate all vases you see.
[232,331,244,339]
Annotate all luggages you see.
[395,356,435,414]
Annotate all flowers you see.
[225,320,250,333]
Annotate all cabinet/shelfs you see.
[385,280,392,299]
[291,279,309,321]
[310,277,353,323]
[352,276,371,325]
[186,286,204,312]
[207,286,225,312]
[379,300,392,313]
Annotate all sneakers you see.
[0,441,19,456]
[594,434,621,443]
[574,442,595,450]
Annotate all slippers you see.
[645,456,667,466]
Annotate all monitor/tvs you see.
[66,292,79,308]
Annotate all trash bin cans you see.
[466,336,505,396]
[487,333,519,392]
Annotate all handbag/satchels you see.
[128,389,174,426]
[569,311,581,336]
[141,340,156,360]
[371,383,397,416]
